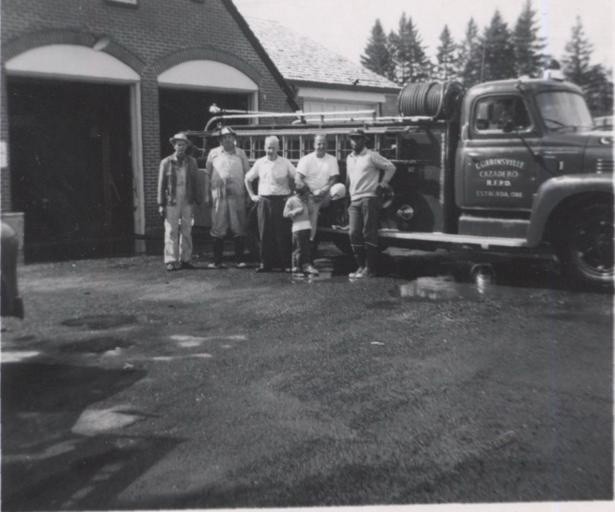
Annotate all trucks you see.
[181,73,615,286]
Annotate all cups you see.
[477,274,492,295]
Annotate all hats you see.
[347,127,370,143]
[219,126,237,137]
[169,133,192,147]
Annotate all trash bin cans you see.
[0,222,24,318]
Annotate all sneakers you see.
[231,258,247,269]
[179,262,193,269]
[208,259,223,270]
[256,264,273,273]
[286,260,321,276]
[167,263,175,271]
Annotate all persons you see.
[155,130,200,272]
[203,125,251,270]
[293,132,345,277]
[281,181,319,276]
[240,134,297,273]
[342,128,398,281]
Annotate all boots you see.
[357,242,381,279]
[330,198,353,231]
[348,242,366,279]
